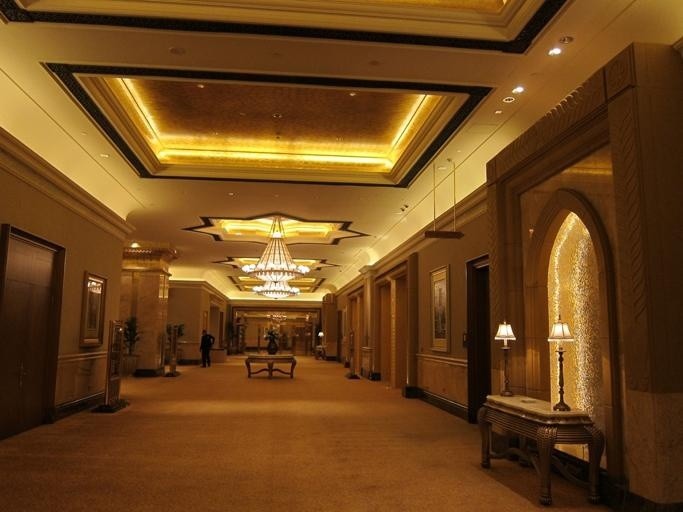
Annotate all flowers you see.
[263,324,282,339]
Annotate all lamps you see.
[547,320,575,411]
[242,219,310,298]
[426,159,464,239]
[318,332,325,345]
[493,322,518,398]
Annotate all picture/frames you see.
[79,270,108,349]
[428,263,449,352]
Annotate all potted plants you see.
[120,316,141,376]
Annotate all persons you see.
[199,329,215,368]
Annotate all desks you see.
[477,394,604,505]
[246,354,297,379]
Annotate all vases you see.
[267,339,278,354]
